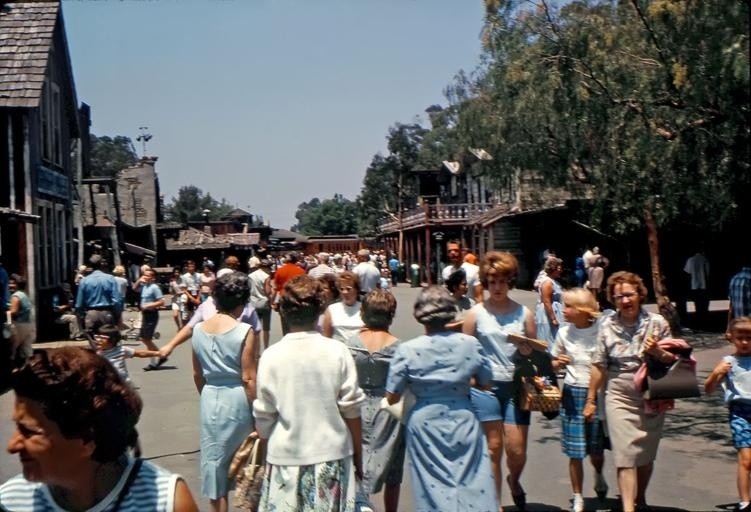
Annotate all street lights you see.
[432,230,445,285]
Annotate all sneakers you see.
[571,491,586,512]
[593,470,609,498]
[142,357,169,371]
[738,501,751,512]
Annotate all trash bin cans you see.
[411,264,421,287]
[398,263,407,283]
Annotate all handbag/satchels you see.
[519,383,563,413]
[642,343,704,400]
